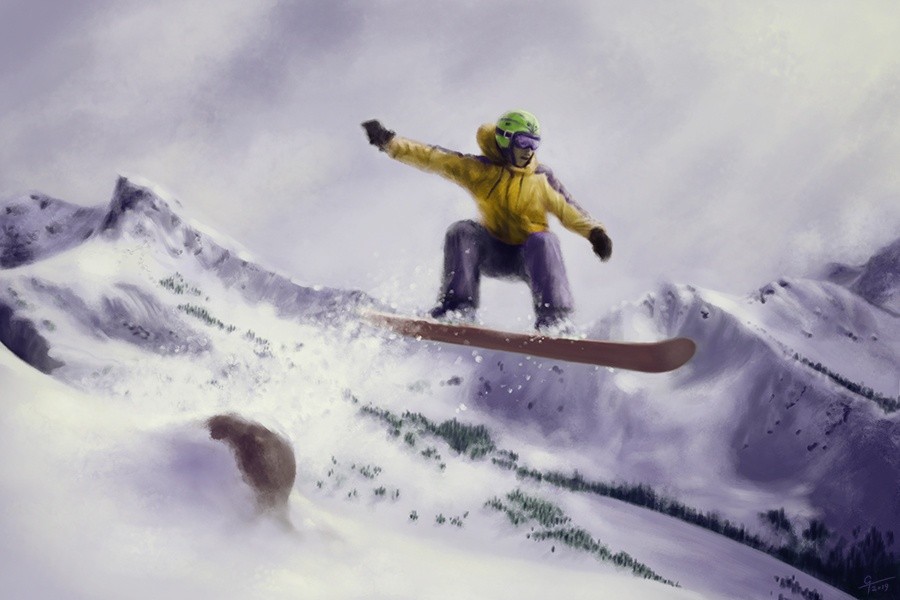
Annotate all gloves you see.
[588,228,613,262]
[362,120,395,149]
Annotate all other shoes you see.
[533,311,574,336]
[426,301,476,324]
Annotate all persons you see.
[359,111,613,339]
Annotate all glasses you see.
[516,136,540,150]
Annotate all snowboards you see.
[370,312,697,374]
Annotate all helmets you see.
[493,109,543,166]
